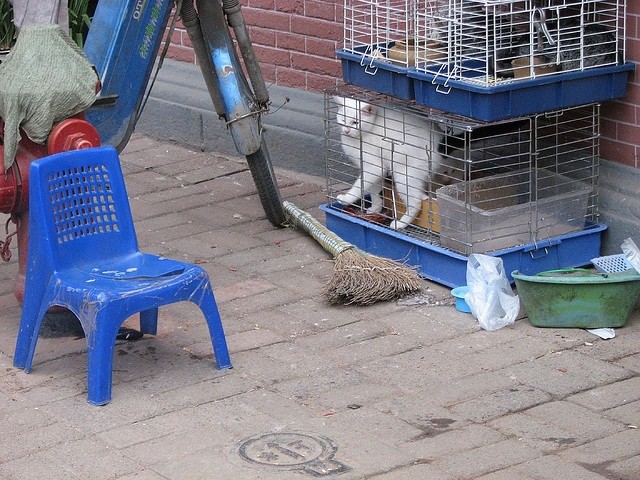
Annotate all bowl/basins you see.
[451,286,471,313]
[341,206,391,222]
[511,57,556,77]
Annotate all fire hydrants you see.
[0,24,100,330]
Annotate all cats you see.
[438,146,478,185]
[331,93,442,231]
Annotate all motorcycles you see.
[82,0,290,229]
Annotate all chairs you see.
[12,145,233,404]
